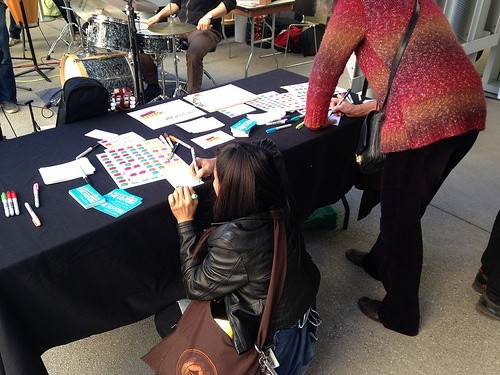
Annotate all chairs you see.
[258,0,318,69]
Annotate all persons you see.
[139,0,237,102]
[9,11,21,46]
[155,138,321,375]
[0,0,18,113]
[304,0,487,336]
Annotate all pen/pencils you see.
[11,191,20,216]
[159,135,173,152]
[169,135,191,149]
[265,119,288,126]
[163,133,174,149]
[79,166,89,183]
[76,143,100,159]
[336,92,354,104]
[169,143,180,159]
[190,147,200,182]
[269,115,291,123]
[191,194,198,199]
[286,113,305,123]
[24,202,41,227]
[265,124,292,133]
[6,191,14,216]
[295,121,305,130]
[33,183,40,208]
[1,192,10,217]
[328,88,352,118]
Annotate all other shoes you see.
[472,267,489,294]
[9,37,21,47]
[475,290,500,321]
[139,83,163,107]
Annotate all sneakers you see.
[1,103,19,114]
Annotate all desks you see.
[222,0,296,77]
[0,67,367,375]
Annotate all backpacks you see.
[56,77,111,128]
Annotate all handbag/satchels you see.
[140,297,266,375]
[273,25,303,53]
[353,109,390,177]
[246,17,271,50]
[297,22,327,58]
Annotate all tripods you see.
[12,2,54,82]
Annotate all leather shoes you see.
[358,297,383,323]
[345,248,370,268]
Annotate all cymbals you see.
[70,0,129,25]
[148,20,198,35]
[102,0,159,12]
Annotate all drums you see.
[124,10,159,31]
[85,13,131,53]
[4,0,39,28]
[59,52,138,108]
[136,29,183,53]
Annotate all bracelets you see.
[206,13,212,18]
